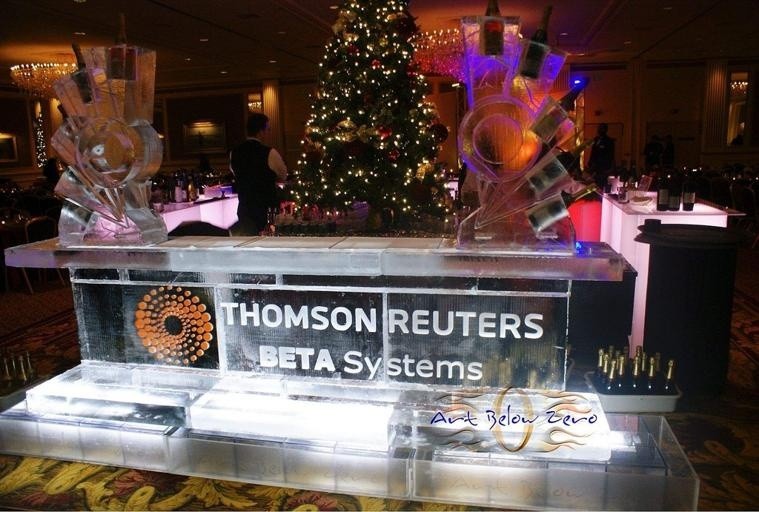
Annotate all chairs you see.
[0,203,69,295]
[664,161,759,250]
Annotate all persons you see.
[584,123,616,187]
[226,112,290,237]
[410,122,448,204]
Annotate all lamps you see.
[9,61,80,103]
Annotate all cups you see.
[617,186,628,203]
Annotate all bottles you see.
[521,4,552,79]
[592,345,674,396]
[153,171,199,213]
[57,103,90,156]
[69,41,94,106]
[527,75,590,143]
[110,13,130,78]
[482,0,504,56]
[526,140,590,198]
[1,351,38,397]
[656,171,697,212]
[619,160,637,183]
[529,182,598,234]
